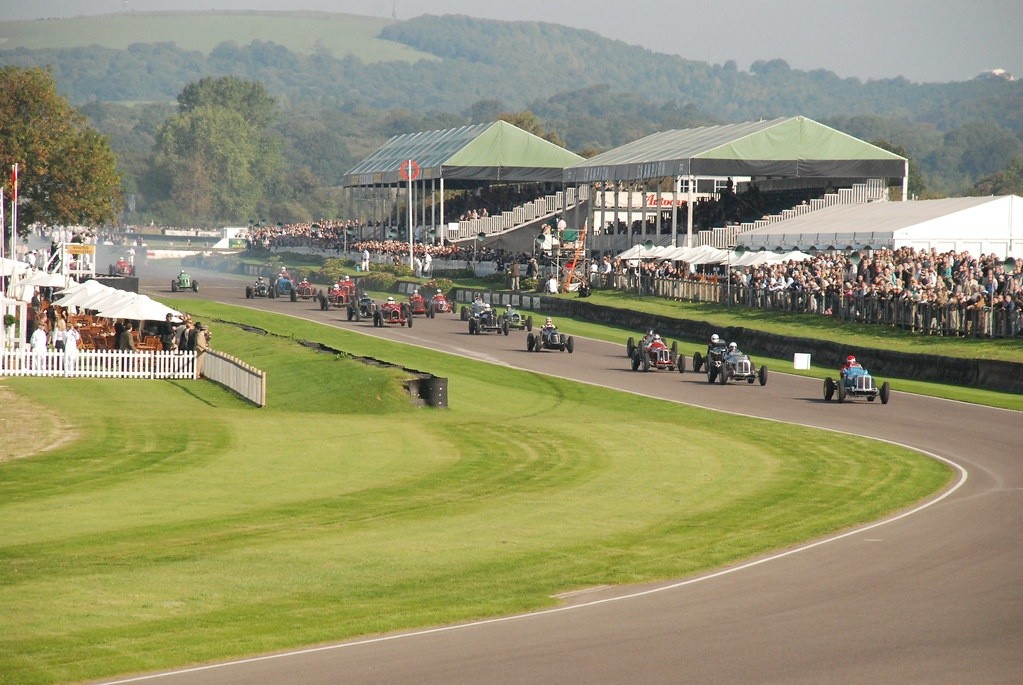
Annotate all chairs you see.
[26,302,162,351]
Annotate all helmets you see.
[729,342,738,350]
[334,284,340,290]
[387,297,393,302]
[647,328,656,336]
[847,355,856,364]
[653,334,661,343]
[414,290,419,295]
[277,274,283,279]
[711,334,720,343]
[437,289,441,293]
[484,304,491,310]
[546,317,552,324]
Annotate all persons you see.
[587,247,1023,380]
[30,221,212,379]
[599,178,835,235]
[245,183,565,339]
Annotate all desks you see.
[137,346,156,350]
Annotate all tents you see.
[342,121,587,244]
[737,194,1022,257]
[562,115,907,247]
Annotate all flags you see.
[11,169,16,201]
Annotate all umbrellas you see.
[0,257,184,338]
[613,243,816,274]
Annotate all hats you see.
[194,322,201,326]
[201,325,208,330]
